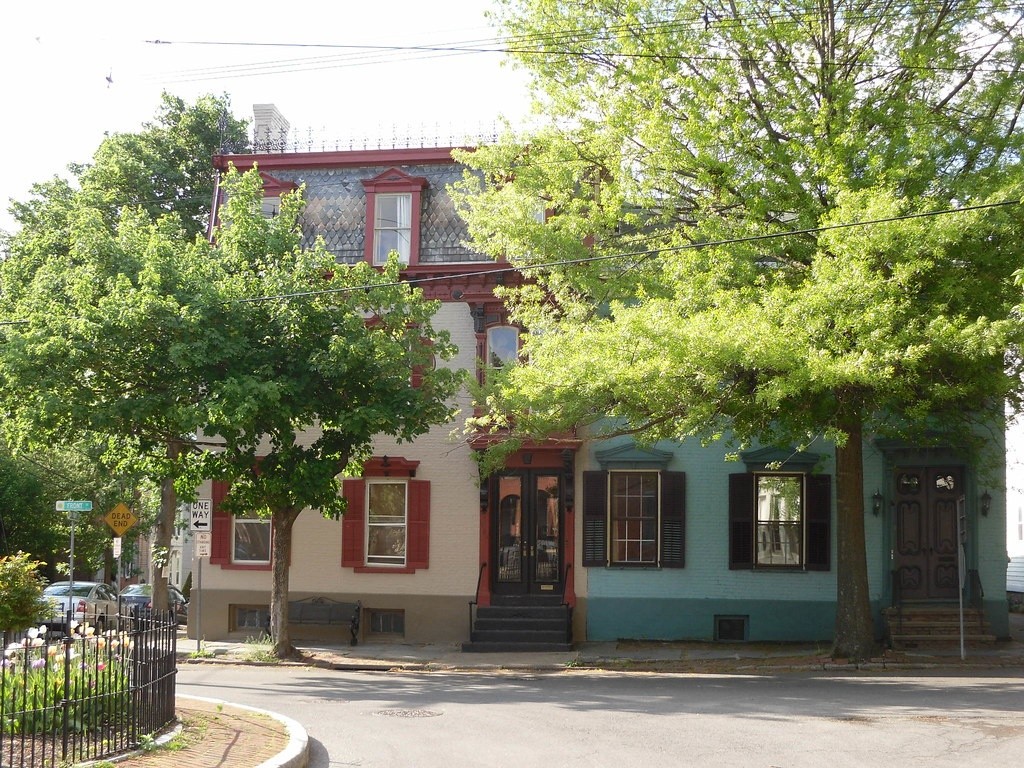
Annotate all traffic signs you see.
[56,501,93,511]
[190,498,212,533]
[68,510,81,522]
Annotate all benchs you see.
[263,595,362,646]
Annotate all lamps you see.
[981,488,992,517]
[872,487,883,516]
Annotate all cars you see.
[36,580,130,635]
[118,584,188,624]
